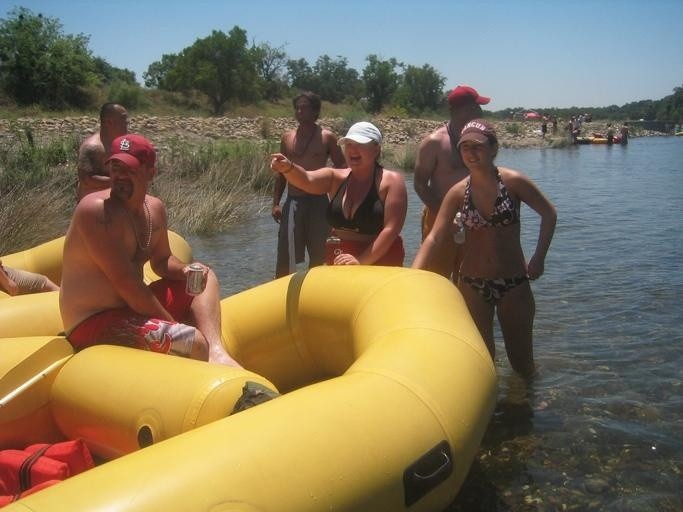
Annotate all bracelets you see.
[279,160,296,175]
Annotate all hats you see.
[448,86,490,106]
[104,135,156,169]
[456,119,497,150]
[337,122,383,148]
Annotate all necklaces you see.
[124,199,153,251]
[291,126,317,157]
[444,122,460,145]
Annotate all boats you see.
[3,229,196,334]
[577,132,610,146]
[675,130,683,137]
[0,263,502,512]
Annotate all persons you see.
[569,115,577,145]
[607,122,614,144]
[540,112,551,139]
[0,259,61,298]
[620,121,629,145]
[77,102,130,204]
[410,118,559,375]
[269,119,407,266]
[552,115,558,135]
[58,133,249,371]
[413,84,492,287]
[270,90,349,281]
[577,114,584,135]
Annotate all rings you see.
[203,266,210,271]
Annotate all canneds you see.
[185,264,204,297]
